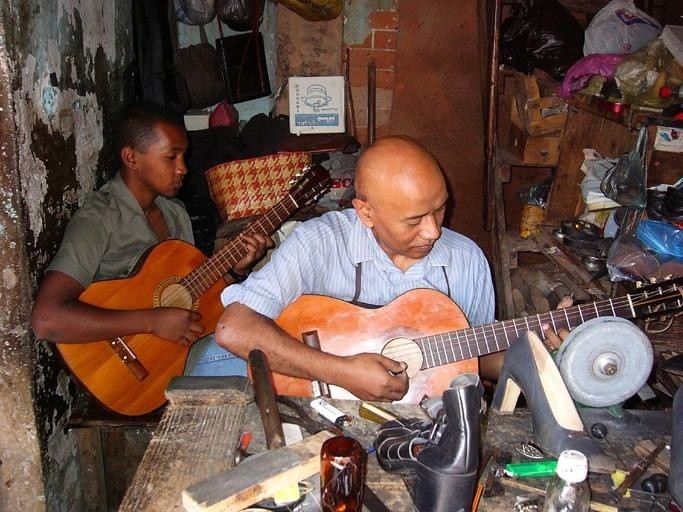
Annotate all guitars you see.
[246,278,682,403]
[52,164,332,418]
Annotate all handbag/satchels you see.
[173,1,271,110]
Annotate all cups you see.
[319,437,366,511]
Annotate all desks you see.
[116,375,670,512]
[542,89,683,227]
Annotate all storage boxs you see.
[274,76,346,135]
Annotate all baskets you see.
[205,151,312,225]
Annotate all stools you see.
[65,391,170,512]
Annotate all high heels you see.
[375,373,484,512]
[492,331,615,474]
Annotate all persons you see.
[209,133,572,405]
[25,98,206,348]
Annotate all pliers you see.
[277,396,345,437]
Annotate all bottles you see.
[658,85,683,101]
[542,449,592,511]
